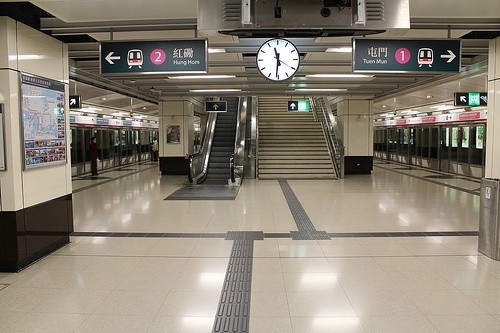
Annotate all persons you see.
[150,135,159,163]
[87,136,101,176]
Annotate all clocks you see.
[256,37,301,81]
